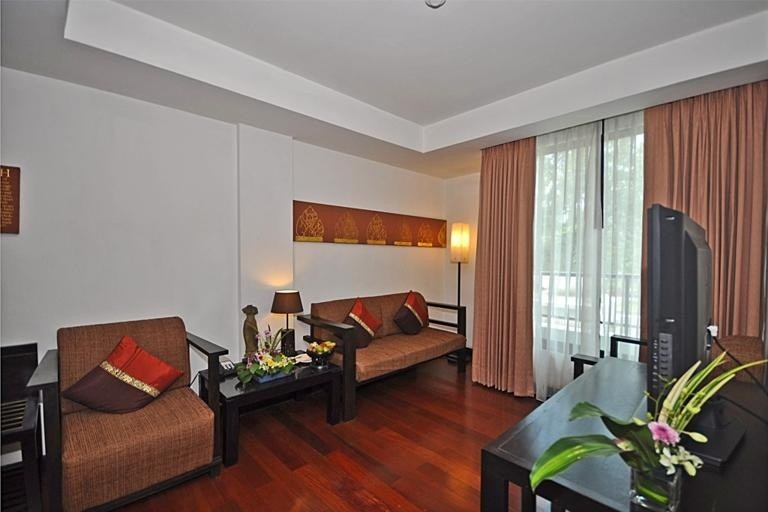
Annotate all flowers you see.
[527,346,767,499]
[235,326,297,387]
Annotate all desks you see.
[478,353,768,512]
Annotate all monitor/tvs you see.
[647,203,746,469]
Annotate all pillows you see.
[59,335,184,413]
[341,295,383,349]
[393,291,428,335]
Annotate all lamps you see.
[446,222,471,364]
[269,288,304,354]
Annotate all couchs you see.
[296,289,469,422]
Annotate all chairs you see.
[24,312,232,512]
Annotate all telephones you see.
[217,355,238,376]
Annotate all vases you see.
[624,457,685,511]
[253,370,290,384]
